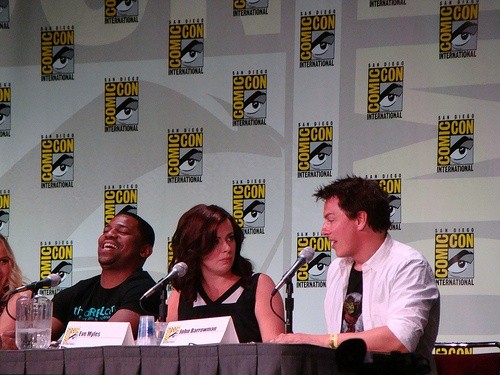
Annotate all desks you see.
[0,342,431,375]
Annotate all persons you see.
[270,176,440,353]
[52,211,161,341]
[166,204,285,343]
[0,233,32,350]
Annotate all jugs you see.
[14,294,54,351]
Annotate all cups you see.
[137,315,168,345]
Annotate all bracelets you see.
[329,333,337,349]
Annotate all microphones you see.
[11,274,61,294]
[271,246,314,296]
[140,262,188,302]
